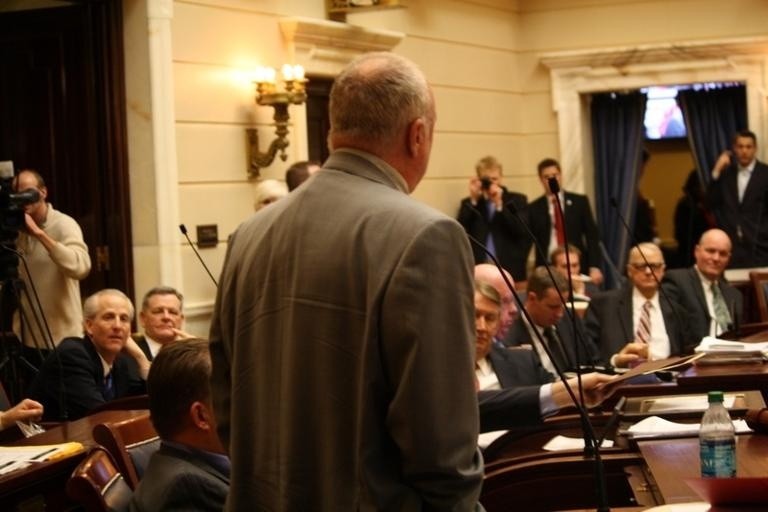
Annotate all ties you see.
[487,199,494,259]
[544,327,570,374]
[630,301,653,369]
[552,198,565,249]
[475,363,480,390]
[106,368,113,399]
[711,284,734,333]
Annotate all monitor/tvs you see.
[642,82,738,139]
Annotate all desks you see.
[3,405,133,510]
[677,328,768,378]
[478,385,768,510]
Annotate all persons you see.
[284,161,322,192]
[46,290,151,411]
[457,156,528,264]
[0,398,44,434]
[703,130,768,269]
[131,337,231,511]
[209,51,484,512]
[550,246,600,292]
[528,159,598,273]
[474,282,648,449]
[505,266,586,379]
[475,263,518,340]
[581,243,697,369]
[660,229,743,355]
[11,168,91,351]
[122,286,191,362]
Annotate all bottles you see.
[699,391,737,478]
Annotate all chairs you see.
[70,445,135,512]
[90,414,161,486]
[749,270,768,327]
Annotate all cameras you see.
[0,161,40,242]
[480,175,491,189]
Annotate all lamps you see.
[245,62,306,178]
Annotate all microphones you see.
[507,200,598,375]
[608,194,684,356]
[179,223,219,288]
[548,174,601,452]
